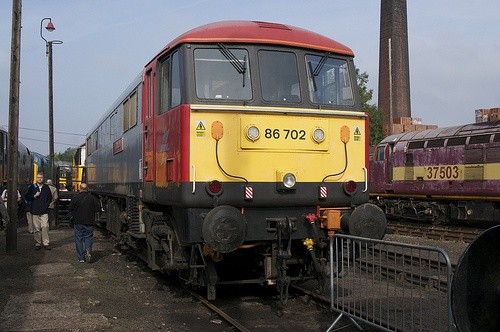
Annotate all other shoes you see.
[78,259,85,262]
[29,231,34,234]
[34,246,40,250]
[85,249,93,263]
[44,246,51,250]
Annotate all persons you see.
[59,184,70,201]
[46,179,58,223]
[26,173,53,249]
[0,178,34,233]
[68,182,100,263]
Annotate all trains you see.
[369,106,500,229]
[0,128,72,196]
[69,18,388,301]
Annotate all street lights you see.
[40,17,65,231]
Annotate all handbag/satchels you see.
[69,216,75,229]
